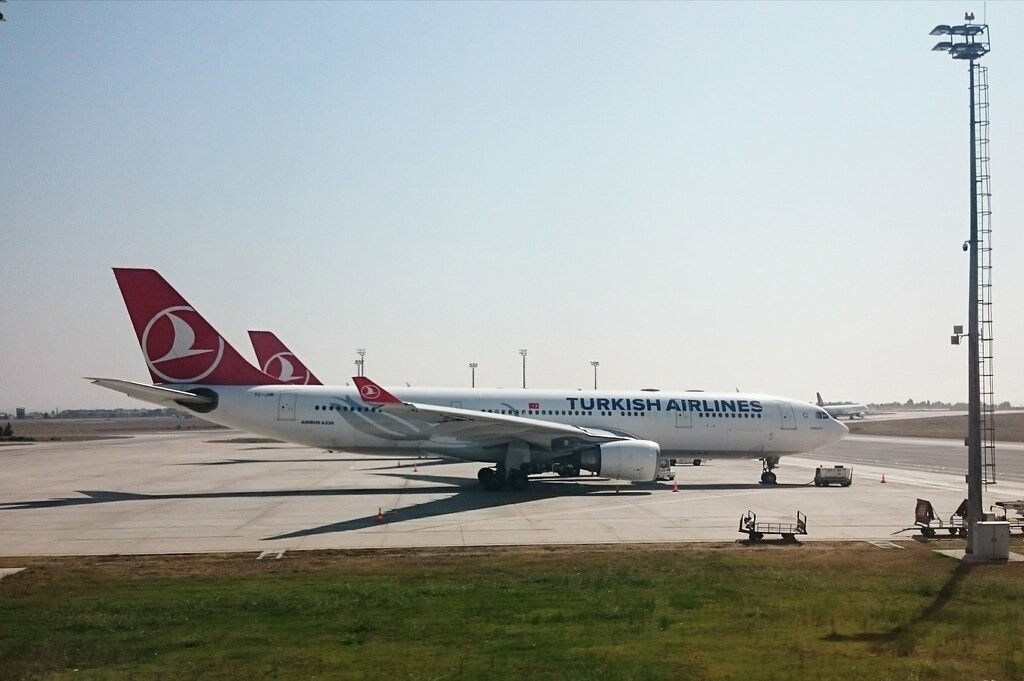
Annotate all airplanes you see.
[80,266,853,495]
[815,392,869,421]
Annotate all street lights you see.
[469,363,478,388]
[519,349,528,389]
[353,348,365,377]
[590,362,600,389]
[928,13,997,555]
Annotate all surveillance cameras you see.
[963,244,968,251]
